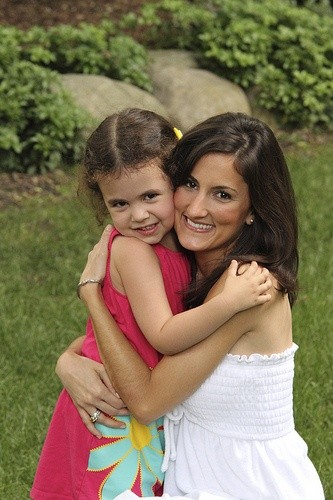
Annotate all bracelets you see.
[75,280,102,299]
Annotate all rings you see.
[89,409,101,424]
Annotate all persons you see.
[27,109,273,500]
[73,109,324,499]
